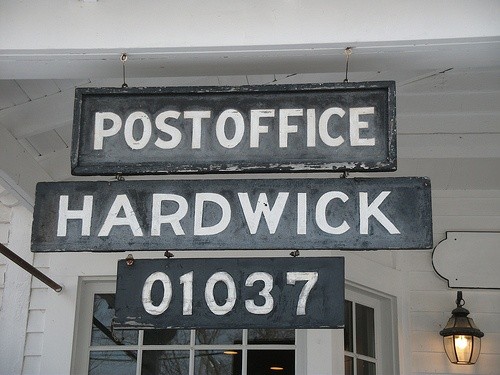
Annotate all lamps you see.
[439,291,483,363]
